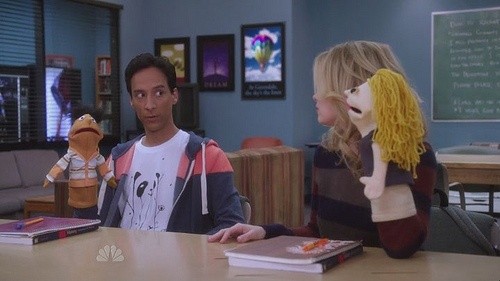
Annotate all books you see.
[224,235,363,274]
[99,59,111,74]
[99,76,113,135]
[0,215,101,246]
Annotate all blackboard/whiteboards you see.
[430,7,500,123]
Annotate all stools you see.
[25,195,54,218]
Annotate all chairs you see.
[431,164,466,210]
[240,136,281,149]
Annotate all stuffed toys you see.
[42,106,118,208]
[345,69,424,223]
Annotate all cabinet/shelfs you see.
[96,55,111,135]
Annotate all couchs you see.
[0,149,61,214]
[225,146,305,225]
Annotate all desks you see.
[436,153,500,215]
[0,226,500,281]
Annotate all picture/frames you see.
[154,36,189,83]
[196,34,235,91]
[240,22,286,100]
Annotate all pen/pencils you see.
[302,238,329,251]
[25,218,44,226]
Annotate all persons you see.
[208,41,437,259]
[70,53,246,235]
